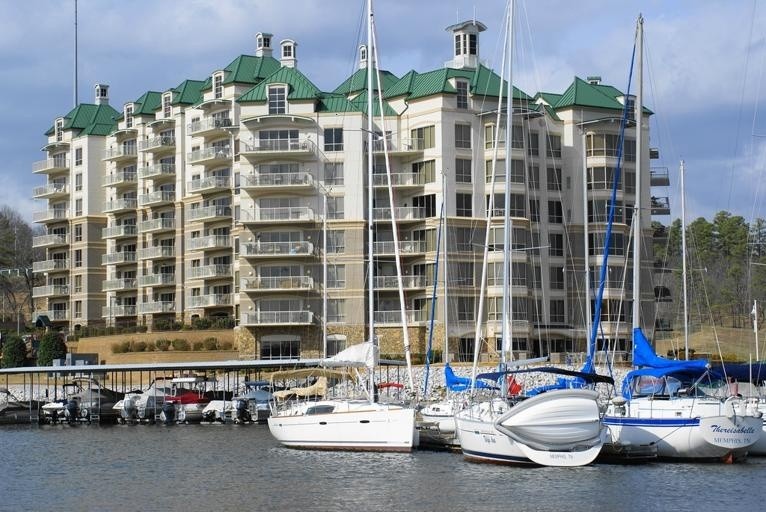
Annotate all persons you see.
[565,355,574,370]
[730,377,742,397]
[85,360,90,374]
[624,350,630,366]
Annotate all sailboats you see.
[267,0,766,467]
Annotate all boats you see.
[0,376,279,425]
